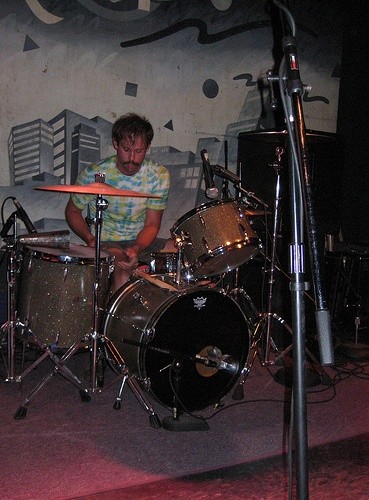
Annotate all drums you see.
[172,199,263,278]
[100,271,252,414]
[15,242,117,350]
[150,251,187,277]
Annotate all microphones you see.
[12,197,37,234]
[200,149,219,199]
[219,355,241,375]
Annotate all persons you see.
[65,112,211,309]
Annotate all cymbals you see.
[35,182,163,200]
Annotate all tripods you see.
[227,137,334,400]
[0,195,161,428]
